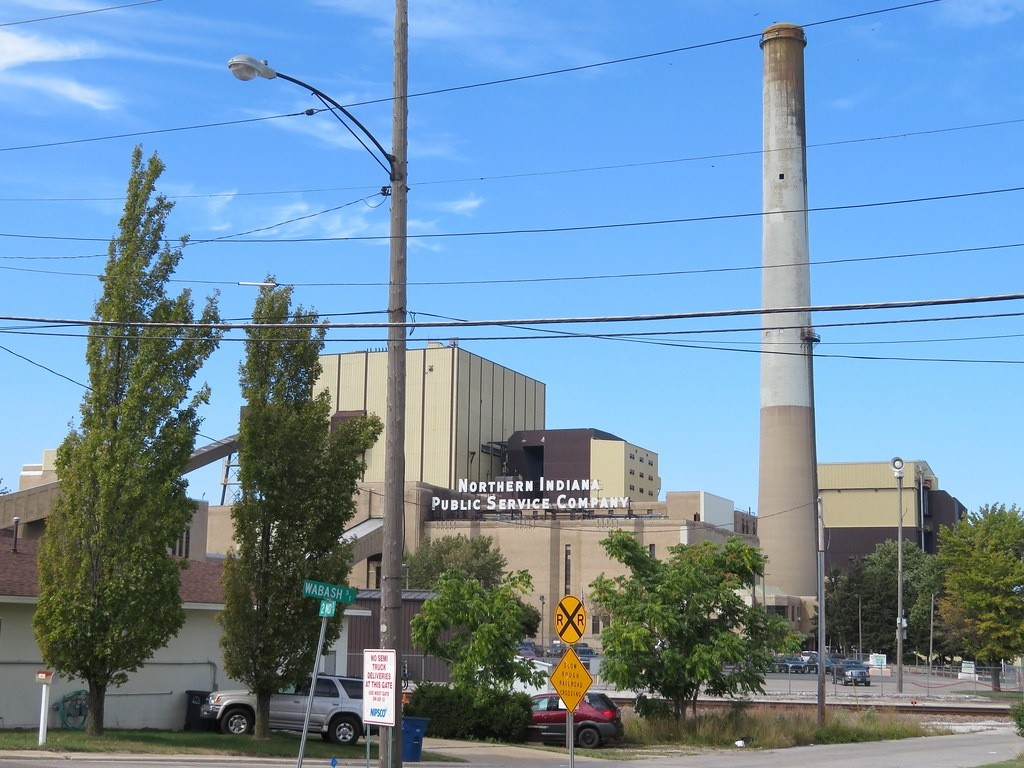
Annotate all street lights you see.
[226,54,410,768]
[540,595,547,648]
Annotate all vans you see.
[817,652,846,672]
[802,650,816,664]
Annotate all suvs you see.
[768,655,806,673]
[200,673,381,745]
[831,659,871,687]
[574,643,599,668]
[528,693,625,750]
[546,644,568,657]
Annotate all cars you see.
[518,641,544,658]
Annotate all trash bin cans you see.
[402,716,431,761]
